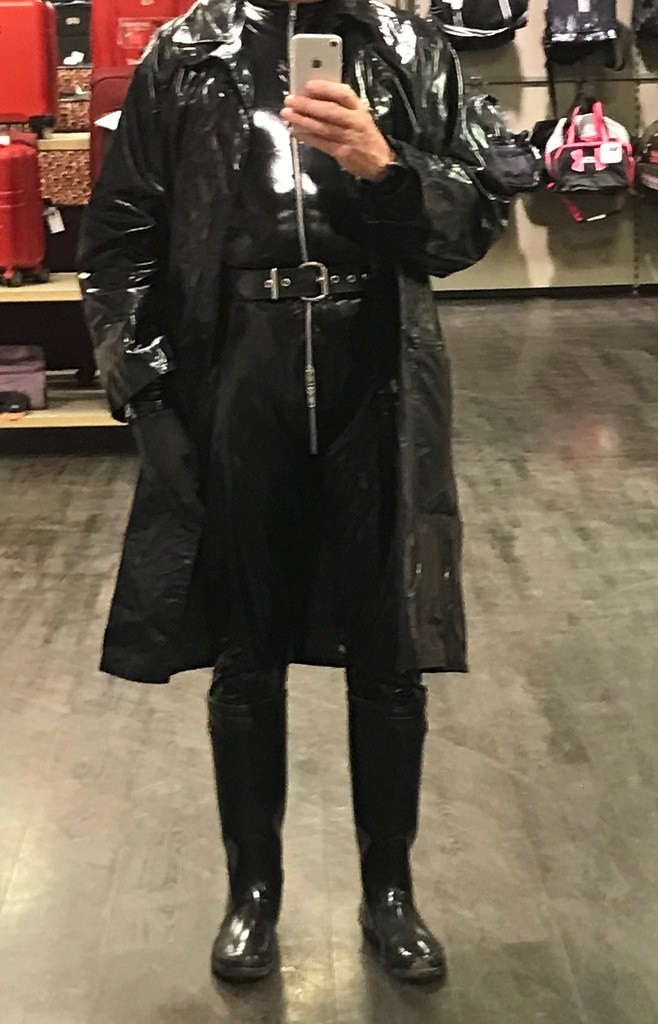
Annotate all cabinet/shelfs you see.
[0,272,131,429]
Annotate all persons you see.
[80,1,509,984]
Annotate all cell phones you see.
[289,33,342,98]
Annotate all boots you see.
[204,687,448,982]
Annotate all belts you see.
[220,263,385,301]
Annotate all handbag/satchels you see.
[530,74,658,223]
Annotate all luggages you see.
[0,0,196,412]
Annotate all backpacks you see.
[460,95,543,199]
[433,0,657,71]
